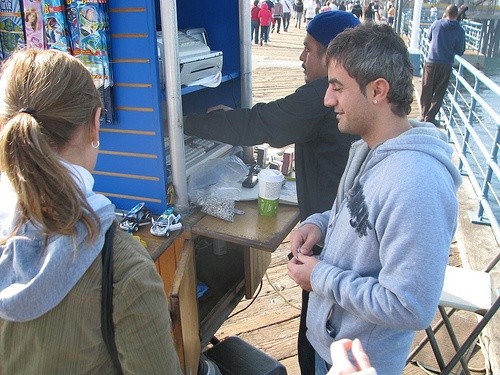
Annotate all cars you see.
[119,202,151,233]
[149,207,183,238]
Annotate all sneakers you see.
[417,116,440,127]
[260,38,267,46]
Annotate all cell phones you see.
[242,174,258,188]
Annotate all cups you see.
[257,168,284,218]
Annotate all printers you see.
[156,30,223,89]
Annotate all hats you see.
[306,10,362,47]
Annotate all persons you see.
[430,4,437,21]
[0,46,184,375]
[183,10,362,375]
[456,4,470,21]
[250,0,261,46]
[262,0,274,12]
[293,0,395,29]
[326,337,377,375]
[282,0,292,32]
[287,24,463,375]
[416,4,466,128]
[258,2,276,46]
[271,0,283,34]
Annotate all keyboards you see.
[164,136,220,176]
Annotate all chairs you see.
[404,247,500,375]
